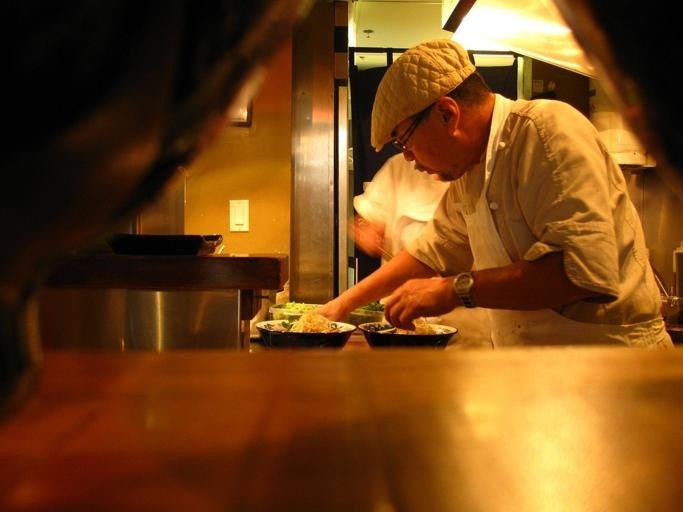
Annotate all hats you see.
[370,39,477,149]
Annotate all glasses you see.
[392,116,421,152]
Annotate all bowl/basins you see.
[358,321,457,352]
[254,319,357,352]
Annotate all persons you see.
[309,37,674,349]
[348,150,451,303]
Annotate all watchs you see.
[452,271,476,308]
